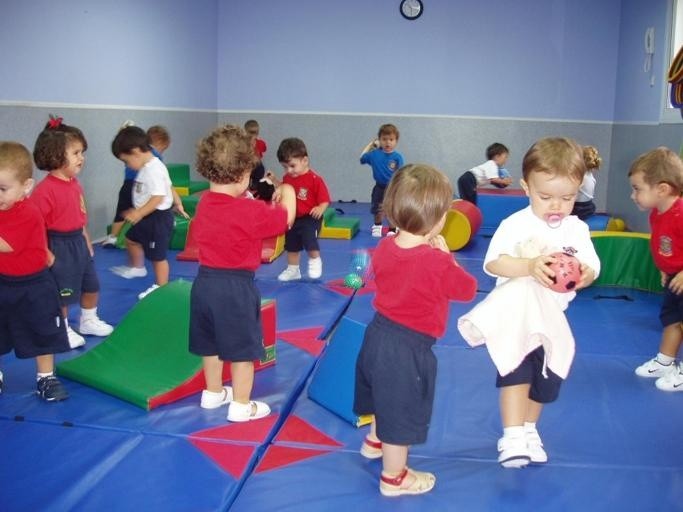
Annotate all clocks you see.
[400,1,424,20]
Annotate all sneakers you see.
[497,428,547,469]
[227,401,272,424]
[199,386,234,409]
[35,234,160,401]
[360,434,385,460]
[635,359,675,378]
[655,361,683,392]
[380,466,437,497]
[277,225,396,281]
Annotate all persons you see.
[265,138,330,282]
[570,145,603,219]
[353,163,478,497]
[458,142,512,208]
[29,115,116,349]
[628,146,683,393]
[101,125,190,246]
[188,124,296,423]
[482,135,602,467]
[359,124,405,238]
[0,142,69,403]
[243,119,266,190]
[112,126,173,301]
